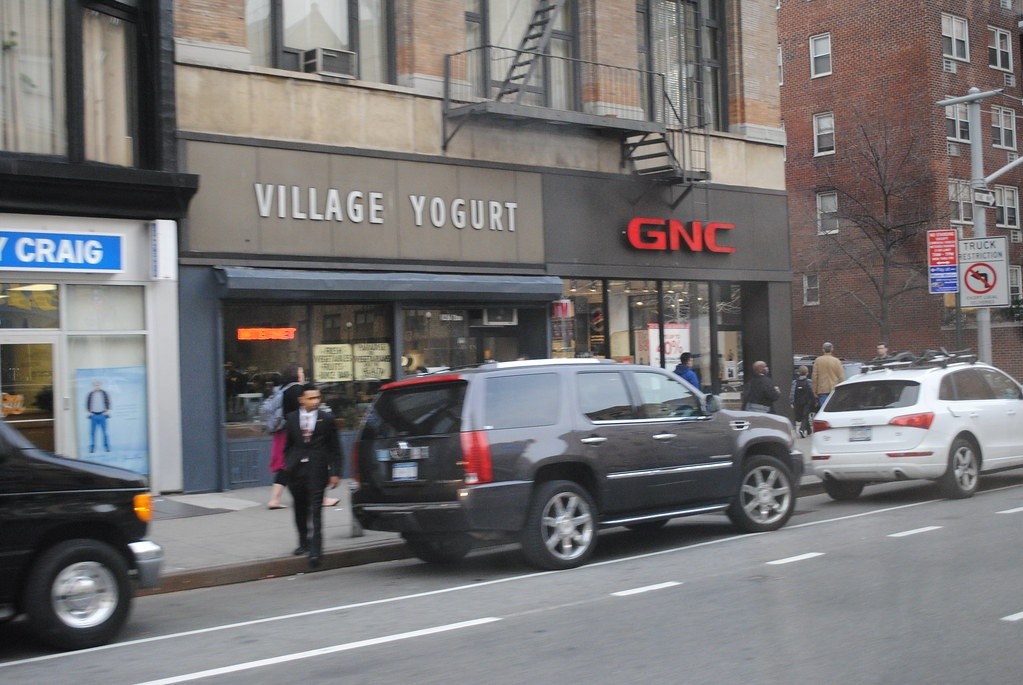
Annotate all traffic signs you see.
[973,188,997,208]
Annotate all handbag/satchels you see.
[746,403,771,413]
[271,429,288,473]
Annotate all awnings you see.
[217,266,563,307]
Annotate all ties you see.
[303,414,312,443]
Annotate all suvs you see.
[352,357,804,571]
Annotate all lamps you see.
[624,281,631,293]
[590,279,598,292]
[642,280,651,291]
[570,279,577,291]
[667,281,675,293]
[682,281,691,293]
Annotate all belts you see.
[91,411,102,415]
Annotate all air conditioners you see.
[469,308,519,327]
[299,46,359,81]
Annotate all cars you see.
[0,420,164,653]
[810,347,1023,503]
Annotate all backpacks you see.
[258,381,302,432]
[794,379,809,404]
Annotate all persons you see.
[86,380,111,453]
[873,342,892,360]
[272,385,344,572]
[790,366,818,438]
[268,363,339,509]
[742,361,782,414]
[812,342,845,410]
[674,352,698,389]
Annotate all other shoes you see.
[322,498,340,507]
[800,430,806,438]
[808,428,813,435]
[90,445,94,452]
[309,553,319,566]
[294,544,312,555]
[268,501,288,508]
[104,444,110,452]
